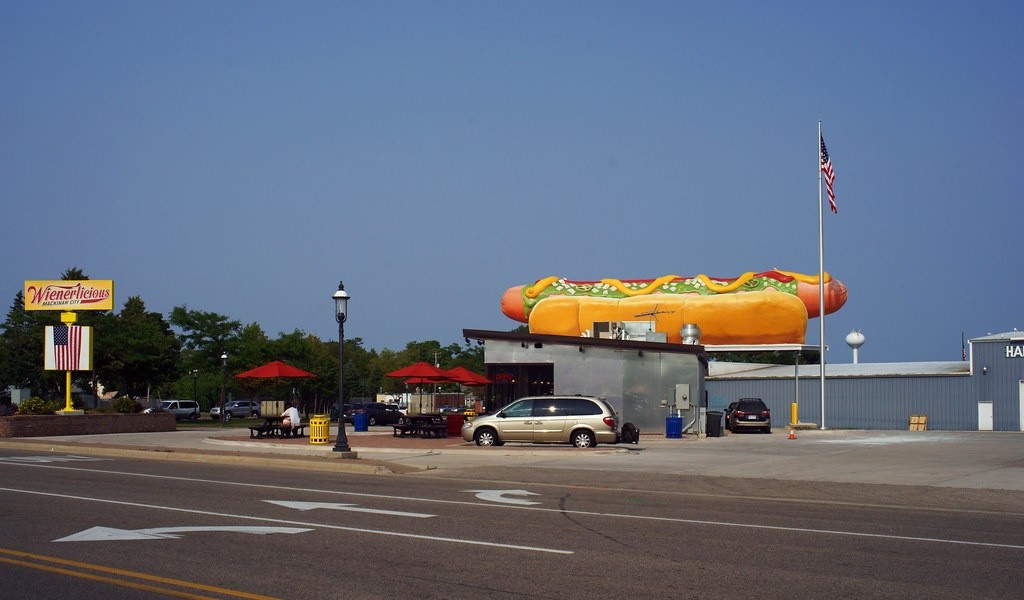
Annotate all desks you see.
[259,415,289,428]
[401,415,435,437]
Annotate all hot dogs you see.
[501,272,847,345]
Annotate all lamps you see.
[579,344,583,352]
[521,341,525,348]
[638,349,642,356]
[465,337,470,343]
[477,339,482,346]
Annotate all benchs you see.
[389,420,444,437]
[248,424,305,437]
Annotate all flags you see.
[53,326,81,371]
[963,345,966,360]
[820,130,838,215]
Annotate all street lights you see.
[192,369,199,423]
[331,280,352,453]
[218,351,229,423]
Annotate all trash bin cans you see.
[706,410,724,436]
[310,415,331,444]
[666,413,682,438]
[355,412,368,431]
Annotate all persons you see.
[281,404,300,435]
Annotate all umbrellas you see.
[384,362,492,414]
[236,360,318,415]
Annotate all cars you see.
[723,398,771,434]
[329,401,407,429]
[437,404,469,413]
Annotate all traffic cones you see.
[788,428,797,440]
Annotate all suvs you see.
[140,399,201,421]
[460,393,620,449]
[209,400,260,422]
